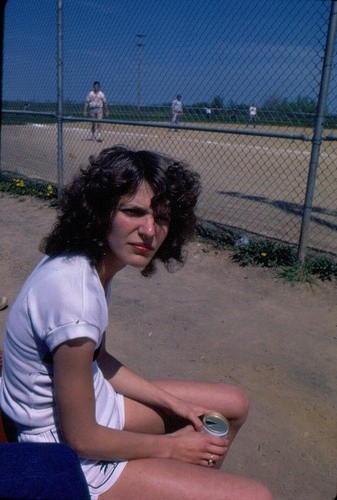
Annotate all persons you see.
[171,95,184,133]
[82,82,110,142]
[246,103,257,128]
[0,145,273,500]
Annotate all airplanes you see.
[133,104,337,123]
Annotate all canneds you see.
[201,412,231,438]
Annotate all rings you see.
[209,454,214,464]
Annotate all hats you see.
[177,95,182,97]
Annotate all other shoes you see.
[96,135,102,140]
[0,296,7,309]
[91,136,95,140]
[175,129,180,132]
